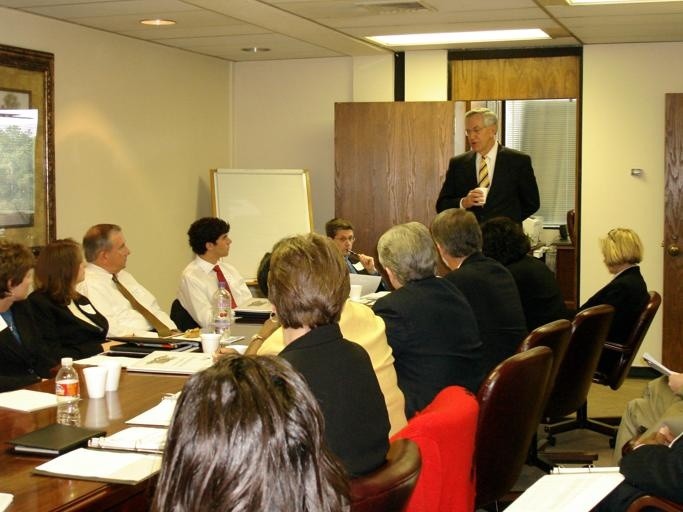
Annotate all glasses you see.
[335,236,356,243]
[607,228,617,246]
[464,127,486,136]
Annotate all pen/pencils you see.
[134,343,178,348]
[345,248,359,258]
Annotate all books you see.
[128,347,217,377]
[0,387,81,414]
[3,423,107,459]
[643,352,673,376]
[233,297,277,319]
[103,334,204,360]
[348,272,391,308]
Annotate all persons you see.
[589,426,683,512]
[75,224,179,338]
[567,228,649,373]
[611,372,683,467]
[0,237,49,394]
[241,251,408,439]
[214,231,391,477]
[150,352,351,512]
[326,216,390,292]
[436,108,540,229]
[26,237,109,363]
[481,215,569,332]
[178,217,252,329]
[431,208,529,365]
[371,221,488,421]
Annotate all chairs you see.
[516,319,599,474]
[477,345,554,510]
[542,304,620,448]
[549,289,661,450]
[388,385,479,512]
[346,437,424,512]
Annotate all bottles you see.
[211,281,231,338]
[55,356,82,428]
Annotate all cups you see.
[473,185,490,204]
[199,331,222,359]
[347,284,365,306]
[82,366,109,400]
[101,361,124,394]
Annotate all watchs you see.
[251,334,265,342]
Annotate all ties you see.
[213,264,237,309]
[112,275,174,337]
[479,156,490,187]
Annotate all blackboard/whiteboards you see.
[211,168,313,285]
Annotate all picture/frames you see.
[1,43,58,258]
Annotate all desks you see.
[1,309,270,512]
